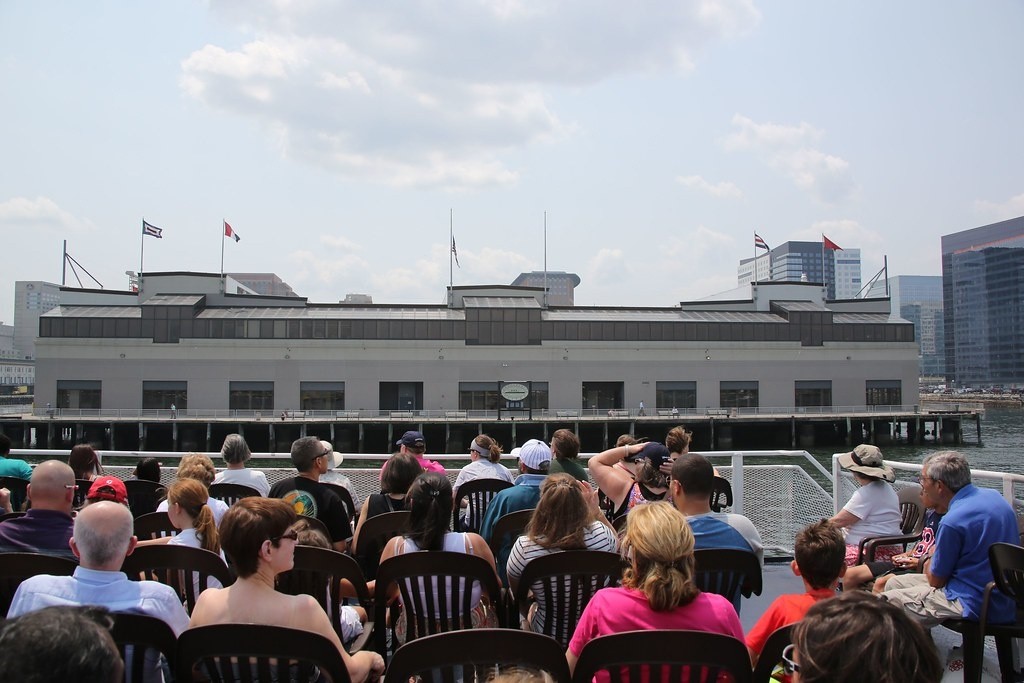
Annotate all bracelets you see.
[594,506,603,521]
[624,444,629,457]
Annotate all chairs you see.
[0,475,1024,683]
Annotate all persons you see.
[0,424,763,683]
[672,406,679,419]
[608,409,615,419]
[170,402,177,420]
[744,516,945,682]
[842,450,1019,627]
[828,443,904,571]
[638,399,647,416]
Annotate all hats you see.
[87,476,129,505]
[837,444,897,483]
[396,431,427,449]
[511,439,553,470]
[319,441,344,470]
[624,442,671,471]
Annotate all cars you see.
[919,386,1024,394]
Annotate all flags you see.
[143,220,163,238]
[224,221,241,242]
[756,234,770,252]
[452,234,461,268]
[824,235,844,250]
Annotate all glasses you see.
[310,447,333,461]
[271,529,299,543]
[666,476,681,487]
[779,645,812,678]
[467,447,481,454]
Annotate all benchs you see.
[444,411,468,419]
[657,410,680,419]
[555,411,579,419]
[390,412,414,419]
[336,412,360,420]
[286,411,306,419]
[608,410,630,417]
[705,409,729,417]
